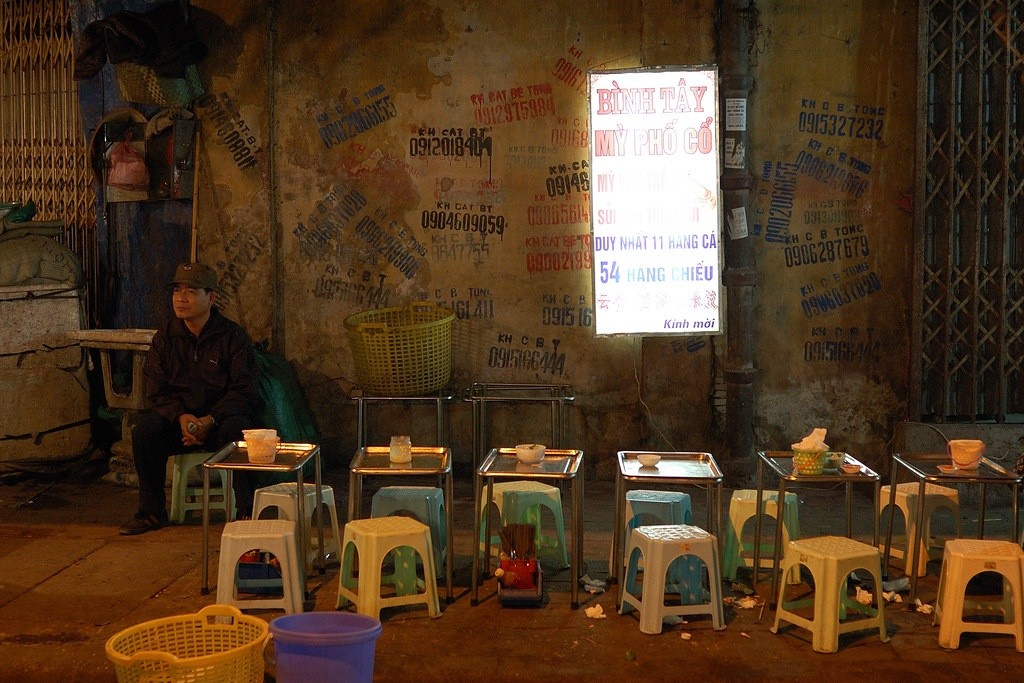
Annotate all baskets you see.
[342,300,456,397]
[106,603,272,683]
[115,62,204,109]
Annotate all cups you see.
[501,558,536,580]
[241,550,259,562]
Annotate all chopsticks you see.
[499,524,535,554]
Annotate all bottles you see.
[495,568,537,590]
[389,436,412,463]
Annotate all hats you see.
[167,264,217,294]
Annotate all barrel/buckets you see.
[269,611,382,683]
[950,440,983,469]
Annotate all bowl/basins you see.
[841,464,862,473]
[824,452,844,471]
[637,455,661,466]
[516,444,545,462]
[937,465,960,473]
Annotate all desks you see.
[201,441,326,599]
[350,446,453,606]
[473,448,584,610]
[884,453,1022,612]
[607,451,724,586]
[756,451,882,623]
[64,327,158,488]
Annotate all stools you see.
[371,487,447,582]
[769,536,891,653]
[335,516,442,619]
[478,481,570,570]
[880,482,960,578]
[931,539,1024,655]
[608,490,703,606]
[253,483,341,569]
[215,519,305,617]
[619,524,727,633]
[726,490,802,587]
[172,454,237,525]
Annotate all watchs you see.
[207,414,218,426]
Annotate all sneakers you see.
[237,511,251,522]
[118,513,168,535]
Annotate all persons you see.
[117,262,269,535]
